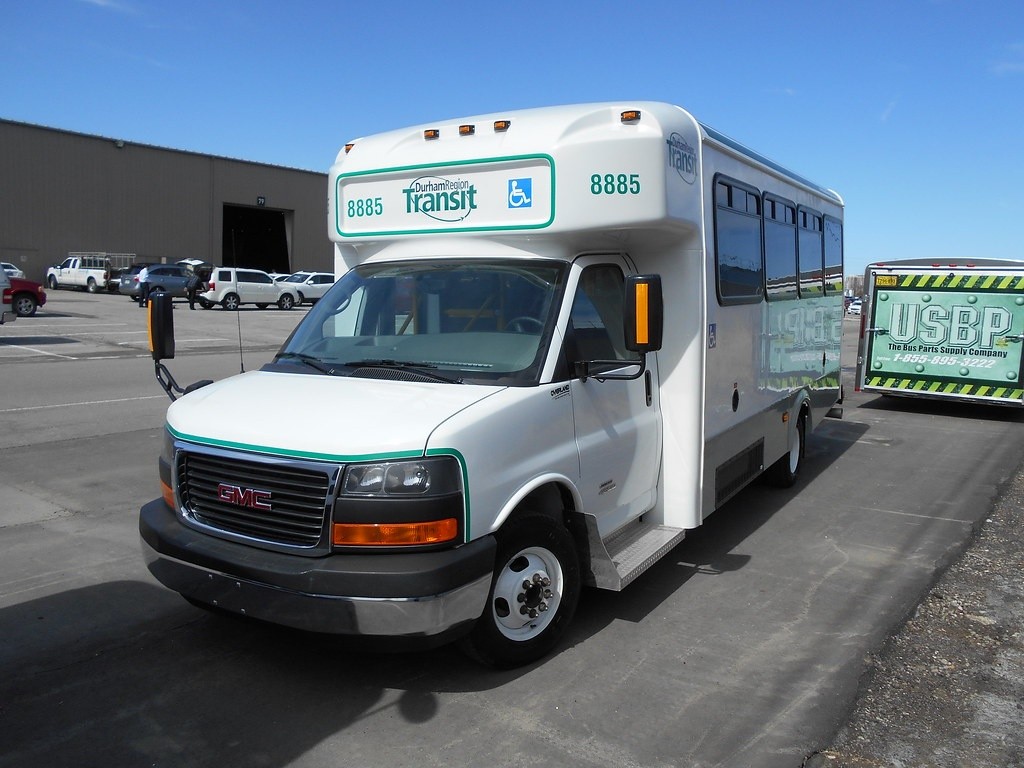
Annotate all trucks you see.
[133,103,847,669]
[852,256,1024,410]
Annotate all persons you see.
[137,264,152,307]
[186,269,207,310]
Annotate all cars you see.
[844,295,862,316]
[269,271,291,282]
[0,262,47,325]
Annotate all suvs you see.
[118,262,205,302]
[184,266,299,311]
[280,269,335,307]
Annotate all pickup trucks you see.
[46,251,137,294]
